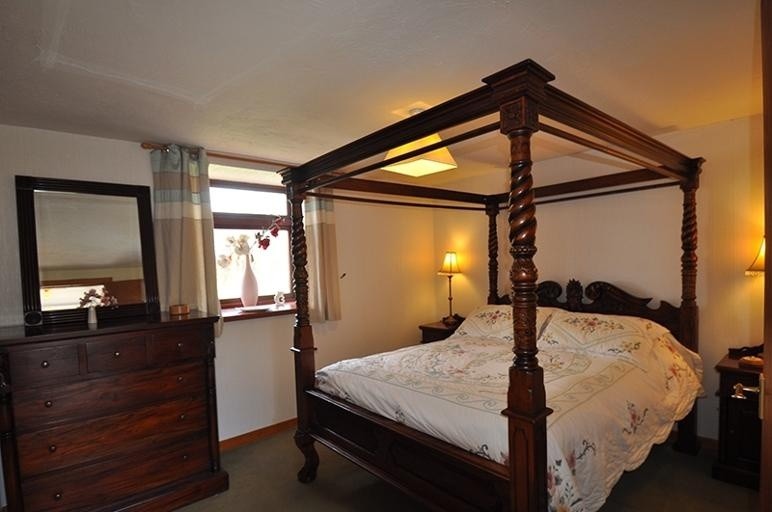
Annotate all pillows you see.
[451,303,551,346]
[535,309,670,373]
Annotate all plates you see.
[241,307,269,312]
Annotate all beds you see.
[275,60,704,512]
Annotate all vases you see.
[88,305,97,324]
[240,255,259,307]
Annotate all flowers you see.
[79,287,120,310]
[217,215,292,269]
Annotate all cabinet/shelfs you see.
[0,308,229,512]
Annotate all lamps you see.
[744,234,766,276]
[378,98,459,180]
[436,252,464,326]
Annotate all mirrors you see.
[15,174,161,326]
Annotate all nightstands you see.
[418,318,466,344]
[713,351,764,492]
